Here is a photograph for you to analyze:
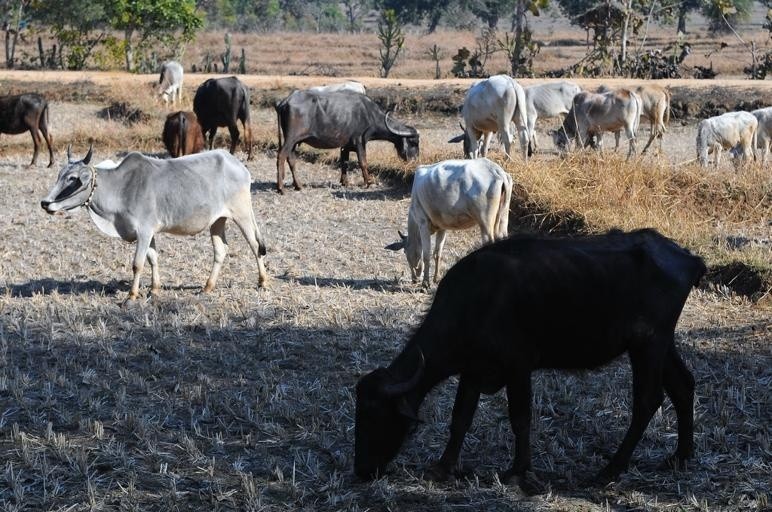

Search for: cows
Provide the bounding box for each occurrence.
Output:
[384,157,513,288]
[354,226,707,488]
[153,61,183,107]
[0,93,55,169]
[274,82,420,194]
[696,106,772,168]
[446,74,532,165]
[40,141,269,309]
[161,111,204,158]
[193,77,256,160]
[497,80,672,163]
